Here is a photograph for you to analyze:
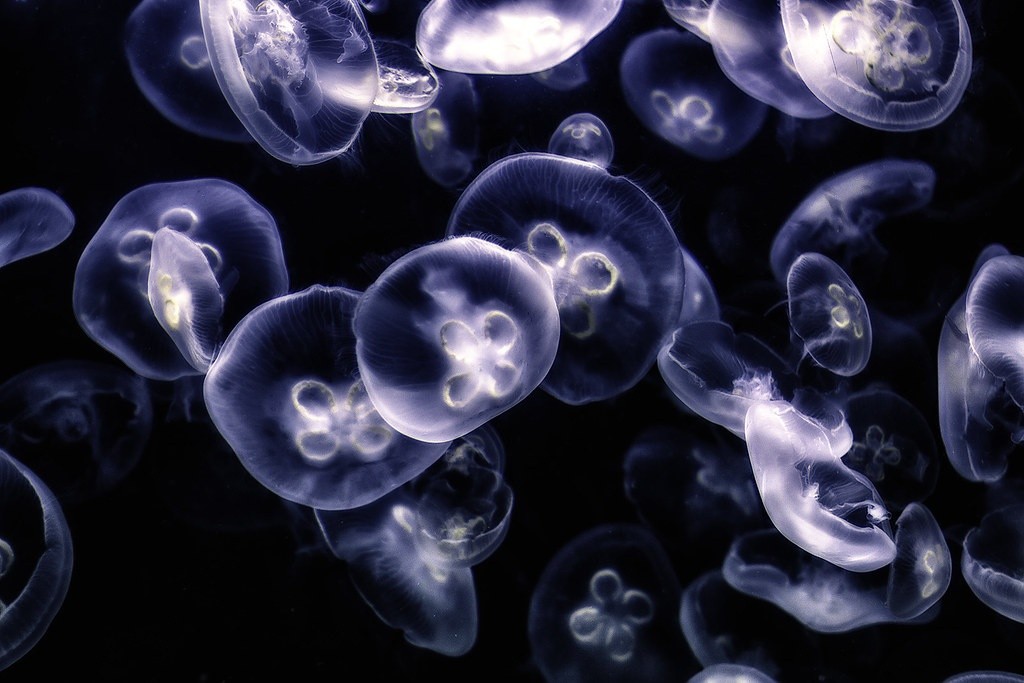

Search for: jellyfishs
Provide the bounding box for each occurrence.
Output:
[0,0,1024,683]
